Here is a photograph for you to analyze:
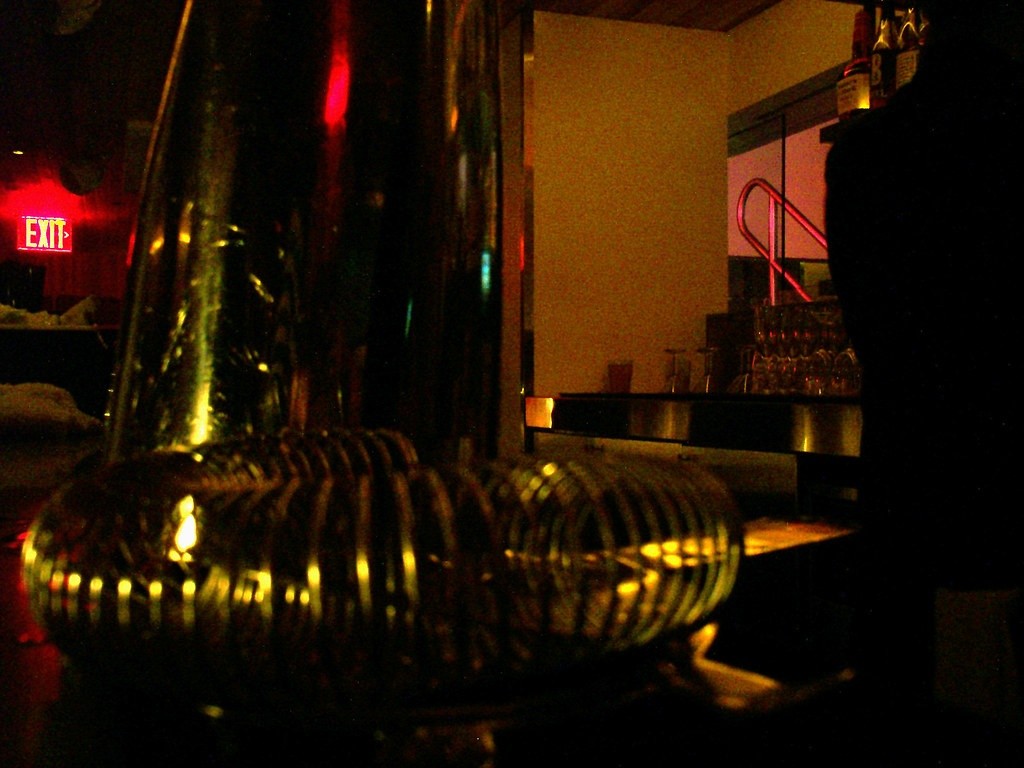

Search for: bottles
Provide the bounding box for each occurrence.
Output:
[743,296,862,395]
[837,0,942,122]
